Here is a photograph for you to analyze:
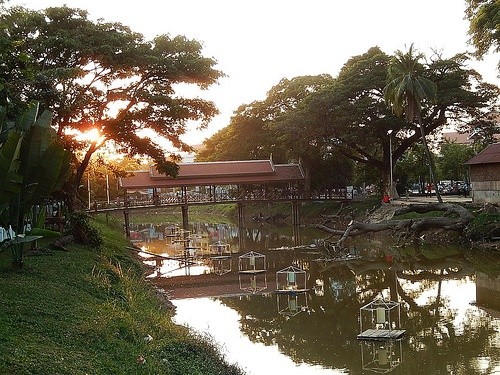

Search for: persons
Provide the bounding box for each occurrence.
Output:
[383,195,389,203]
[117,196,119,204]
[141,194,147,206]
[449,184,451,189]
[127,196,130,201]
[157,194,159,203]
